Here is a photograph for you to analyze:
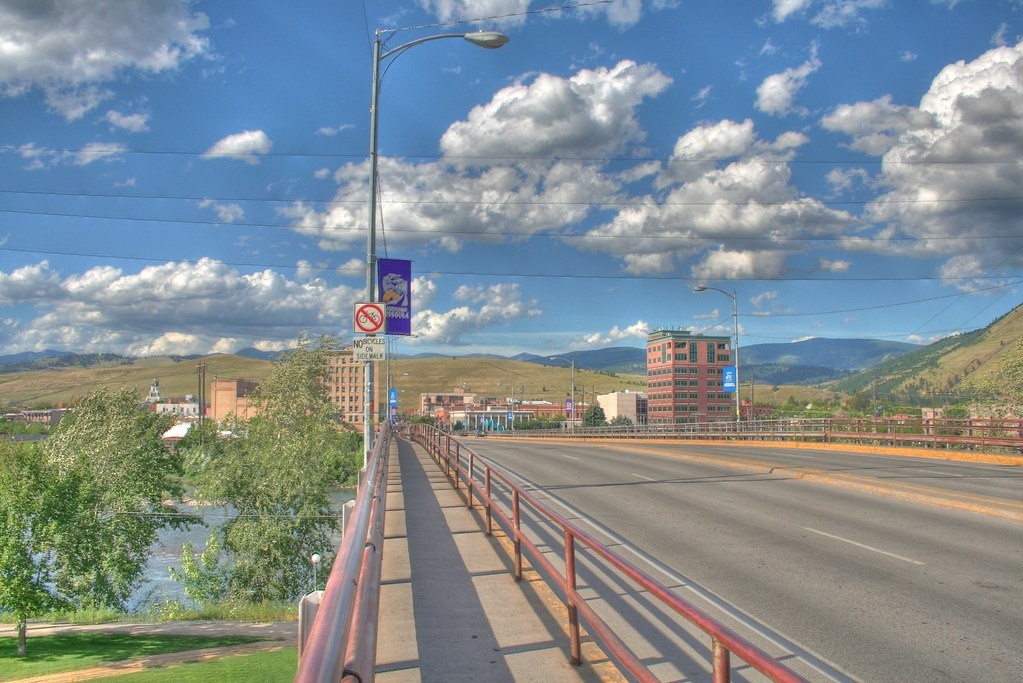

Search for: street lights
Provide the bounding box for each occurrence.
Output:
[497,383,513,431]
[387,334,419,419]
[389,373,410,422]
[550,356,575,434]
[693,286,740,433]
[362,30,509,469]
